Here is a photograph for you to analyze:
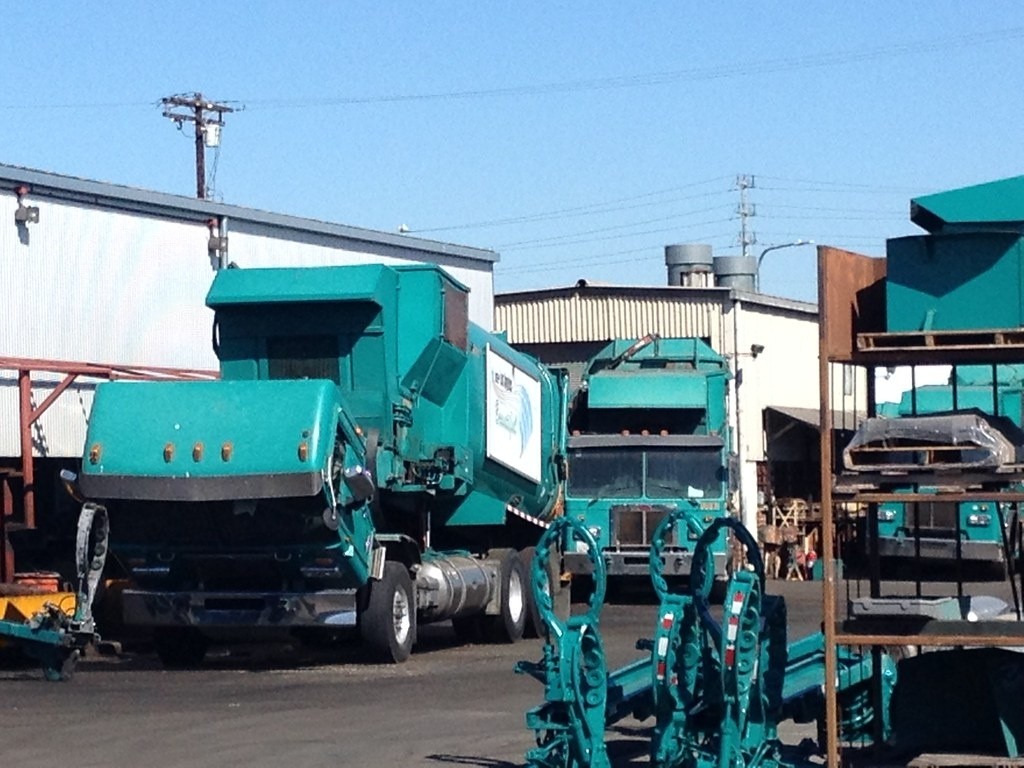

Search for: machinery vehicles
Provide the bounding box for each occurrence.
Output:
[59,262,573,666]
[563,334,744,602]
[832,383,1024,581]
